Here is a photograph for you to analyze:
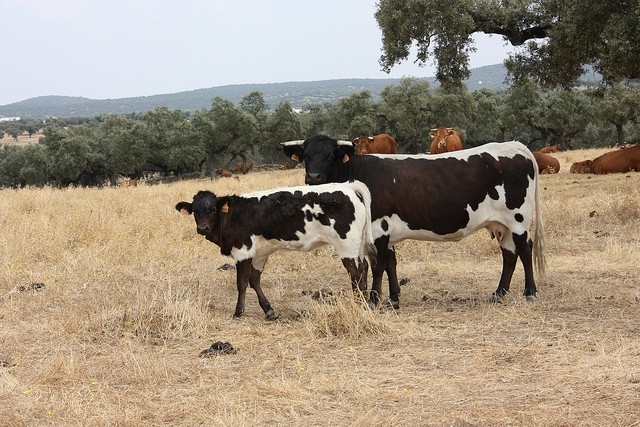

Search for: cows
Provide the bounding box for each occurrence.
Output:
[570,160,593,174]
[541,145,561,154]
[428,127,463,155]
[216,168,232,178]
[174,179,379,322]
[352,133,397,156]
[278,134,550,312]
[591,144,640,174]
[532,151,560,175]
[237,162,254,175]
